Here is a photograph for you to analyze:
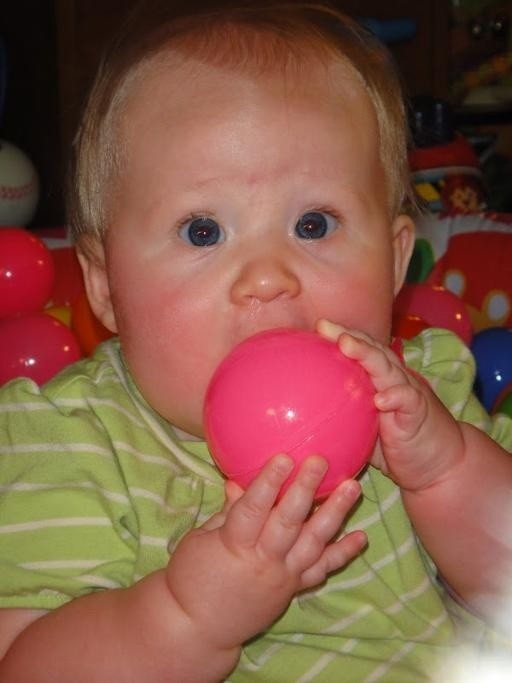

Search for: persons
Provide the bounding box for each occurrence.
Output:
[0,0,511,683]
